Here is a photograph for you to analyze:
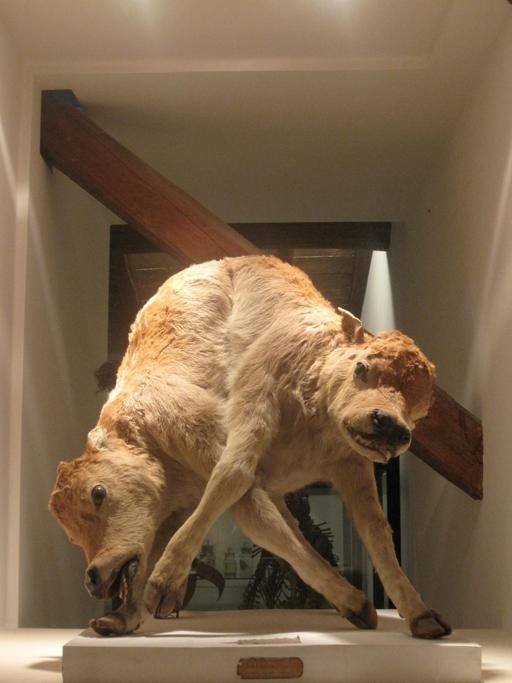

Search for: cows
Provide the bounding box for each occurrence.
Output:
[47,254,451,639]
